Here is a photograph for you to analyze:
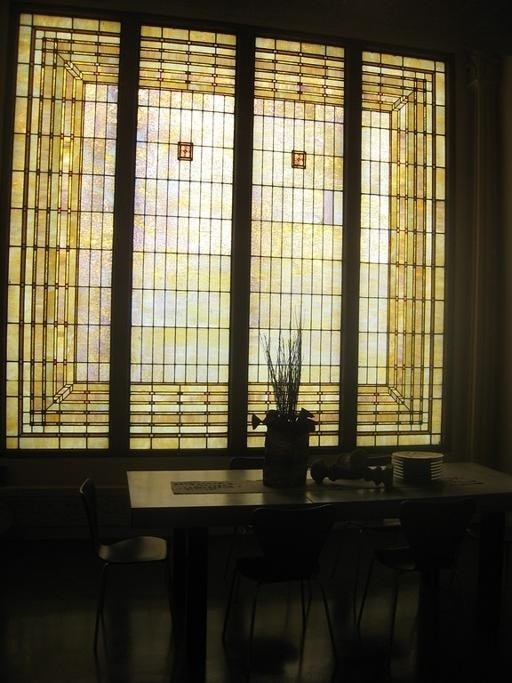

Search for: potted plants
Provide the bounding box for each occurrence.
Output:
[251,321,316,491]
